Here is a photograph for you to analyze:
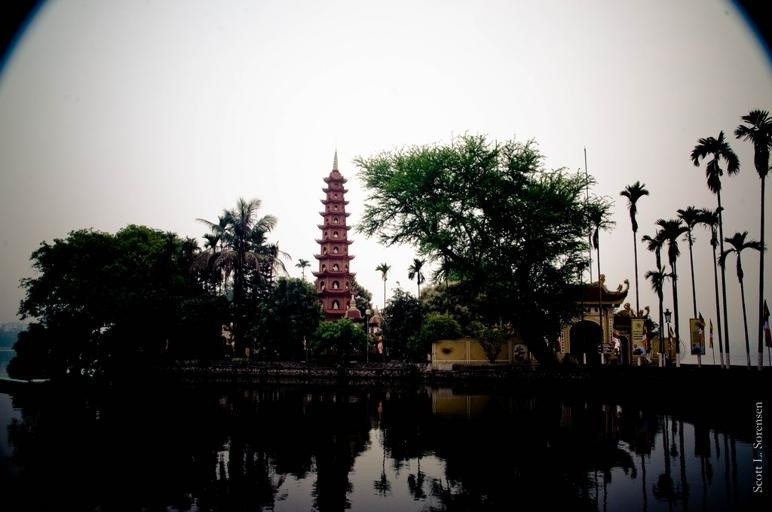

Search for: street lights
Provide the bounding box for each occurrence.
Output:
[364,308,373,367]
[663,308,675,366]
